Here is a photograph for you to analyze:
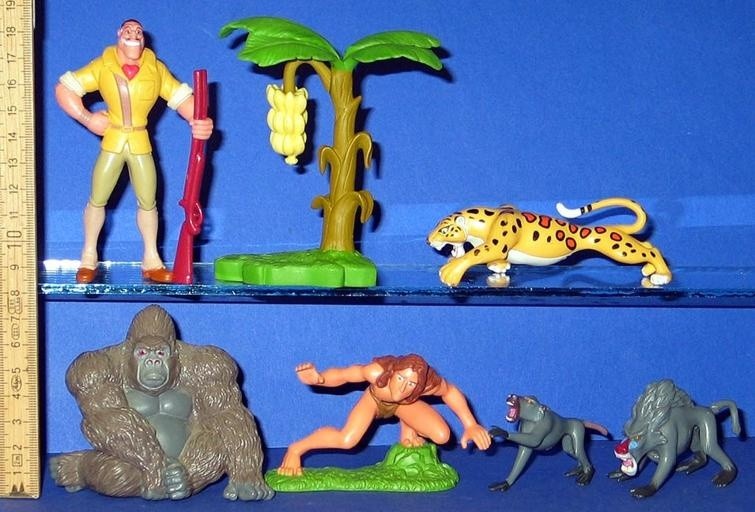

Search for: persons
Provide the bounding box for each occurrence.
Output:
[54,16,213,287]
[261,354,495,495]
